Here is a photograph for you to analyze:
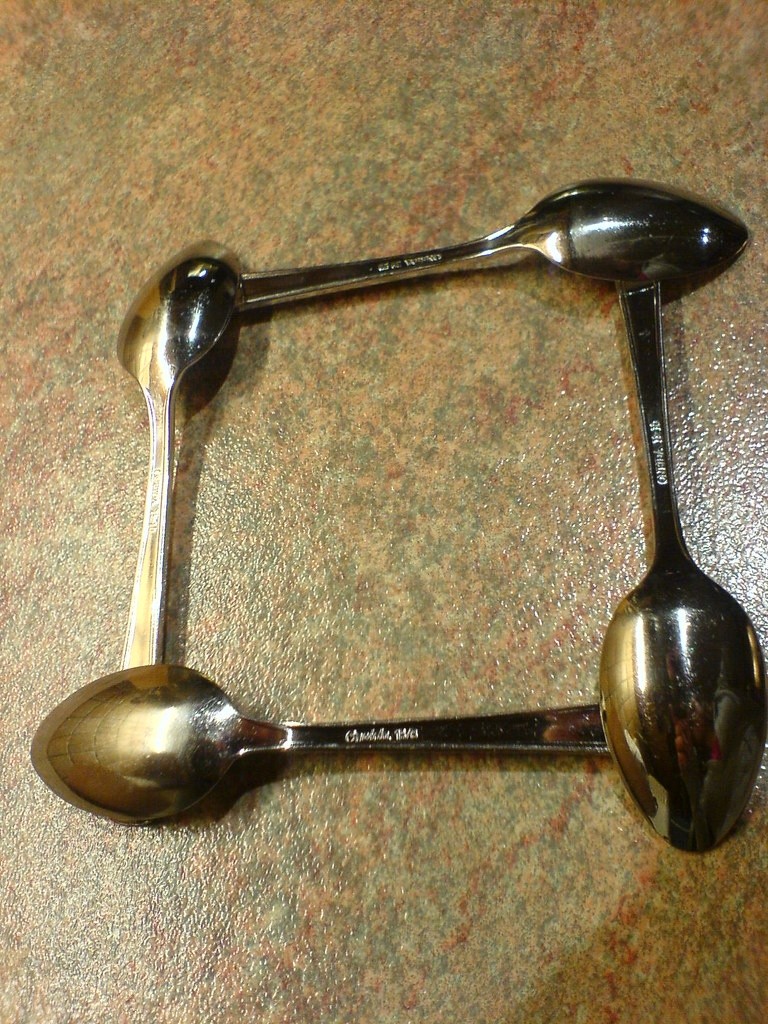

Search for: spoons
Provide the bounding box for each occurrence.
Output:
[115,237,243,673]
[239,178,752,313]
[28,663,604,826]
[594,278,768,852]
